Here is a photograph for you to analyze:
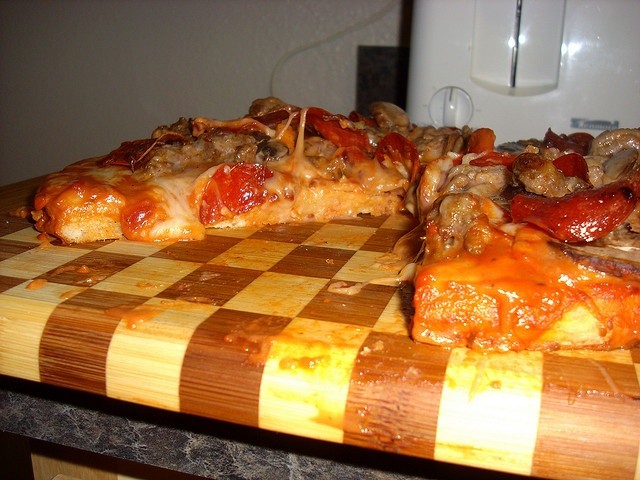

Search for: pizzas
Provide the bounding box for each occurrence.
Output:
[7,96,639,352]
